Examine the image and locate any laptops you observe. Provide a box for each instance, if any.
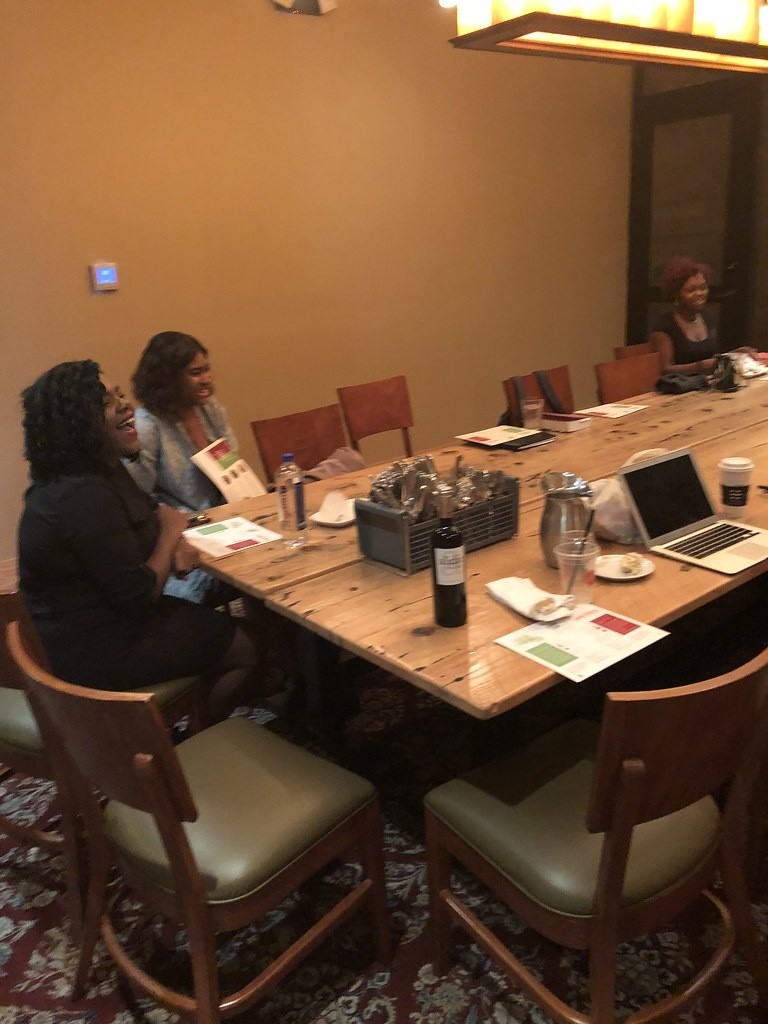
[618,447,768,575]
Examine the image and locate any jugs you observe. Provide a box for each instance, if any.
[539,472,593,569]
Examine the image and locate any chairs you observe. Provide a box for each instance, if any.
[593,352,665,404]
[420,654,768,1023]
[613,342,665,393]
[337,375,418,469]
[248,404,346,494]
[8,606,393,1024]
[501,365,576,430]
[0,592,203,948]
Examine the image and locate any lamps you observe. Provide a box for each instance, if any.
[437,1,767,76]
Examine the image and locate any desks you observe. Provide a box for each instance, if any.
[176,360,767,972]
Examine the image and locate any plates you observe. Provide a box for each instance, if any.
[596,555,655,580]
[309,498,370,528]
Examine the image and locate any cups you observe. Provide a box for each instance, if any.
[717,457,754,517]
[553,542,600,603]
[560,530,593,544]
[521,399,544,430]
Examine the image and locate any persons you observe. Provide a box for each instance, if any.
[650,257,757,376]
[119,329,245,610]
[16,359,254,742]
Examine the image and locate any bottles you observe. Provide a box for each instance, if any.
[276,454,307,547]
[432,492,467,628]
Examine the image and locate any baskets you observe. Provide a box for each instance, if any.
[355,471,520,572]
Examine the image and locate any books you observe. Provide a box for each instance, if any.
[470,424,557,450]
